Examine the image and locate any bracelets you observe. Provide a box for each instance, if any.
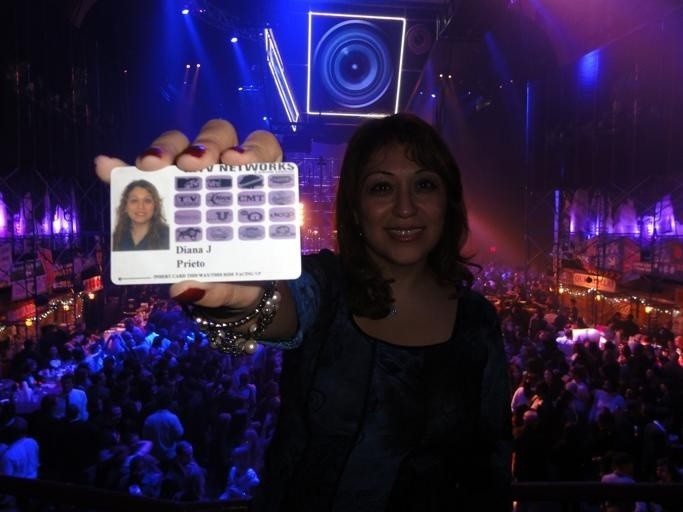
[176,277,282,361]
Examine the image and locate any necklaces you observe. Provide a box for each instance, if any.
[383,278,418,317]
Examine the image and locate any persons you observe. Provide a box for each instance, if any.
[111,169,171,253]
[90,103,518,511]
